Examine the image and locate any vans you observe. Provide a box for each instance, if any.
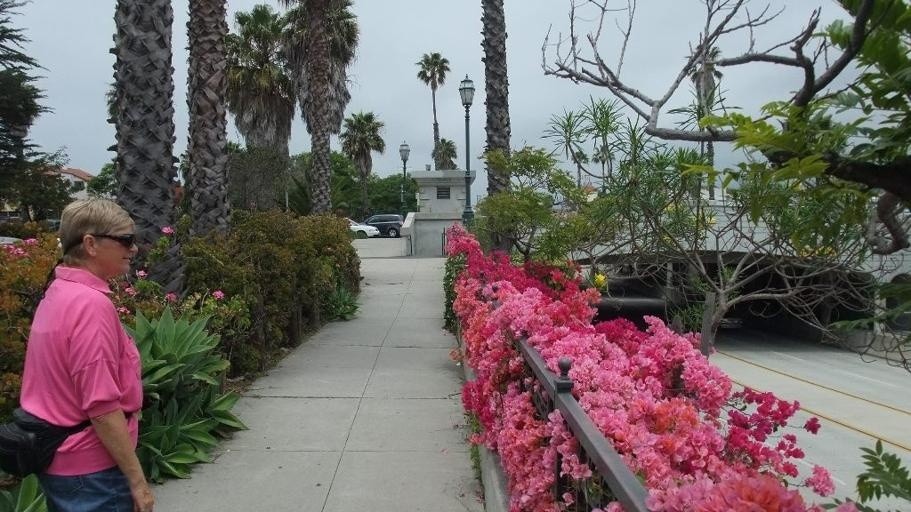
[363,214,405,238]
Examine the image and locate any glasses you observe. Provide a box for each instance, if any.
[80,233,136,248]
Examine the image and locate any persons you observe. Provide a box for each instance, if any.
[20,201,155,512]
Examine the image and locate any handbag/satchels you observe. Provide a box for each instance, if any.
[0,405,70,479]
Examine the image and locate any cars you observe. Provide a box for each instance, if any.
[343,214,380,239]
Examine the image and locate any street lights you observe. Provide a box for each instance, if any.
[460,73,478,235]
[399,139,411,217]
